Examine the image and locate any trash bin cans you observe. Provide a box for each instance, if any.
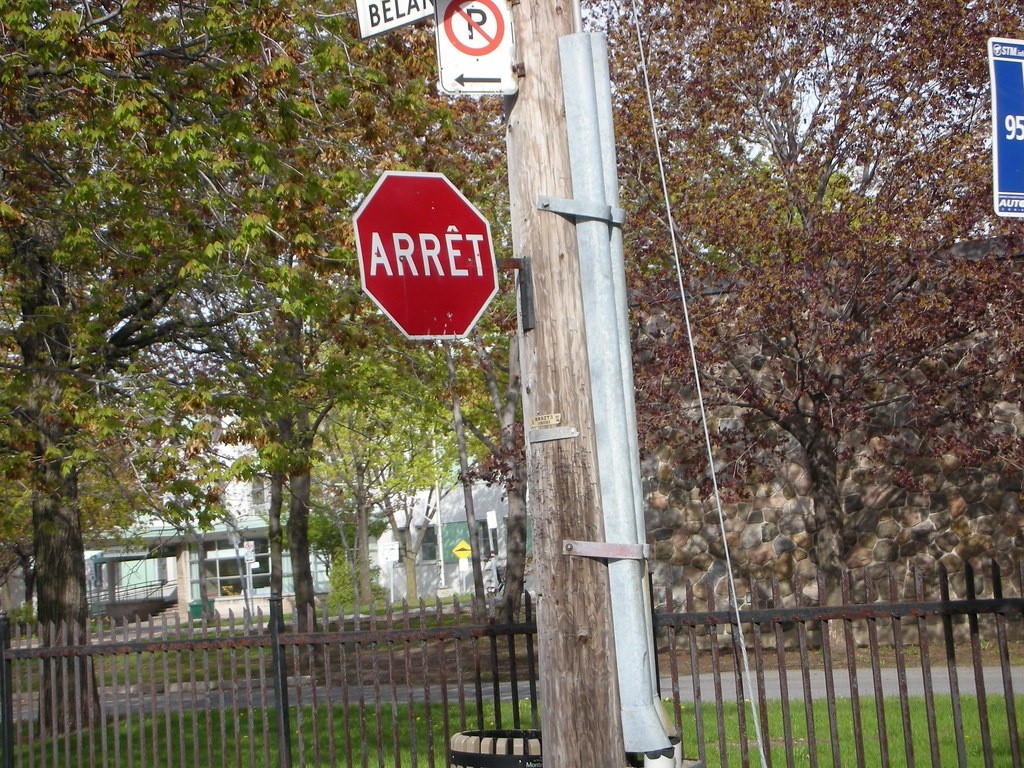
[191,599,215,617]
[449,729,542,768]
